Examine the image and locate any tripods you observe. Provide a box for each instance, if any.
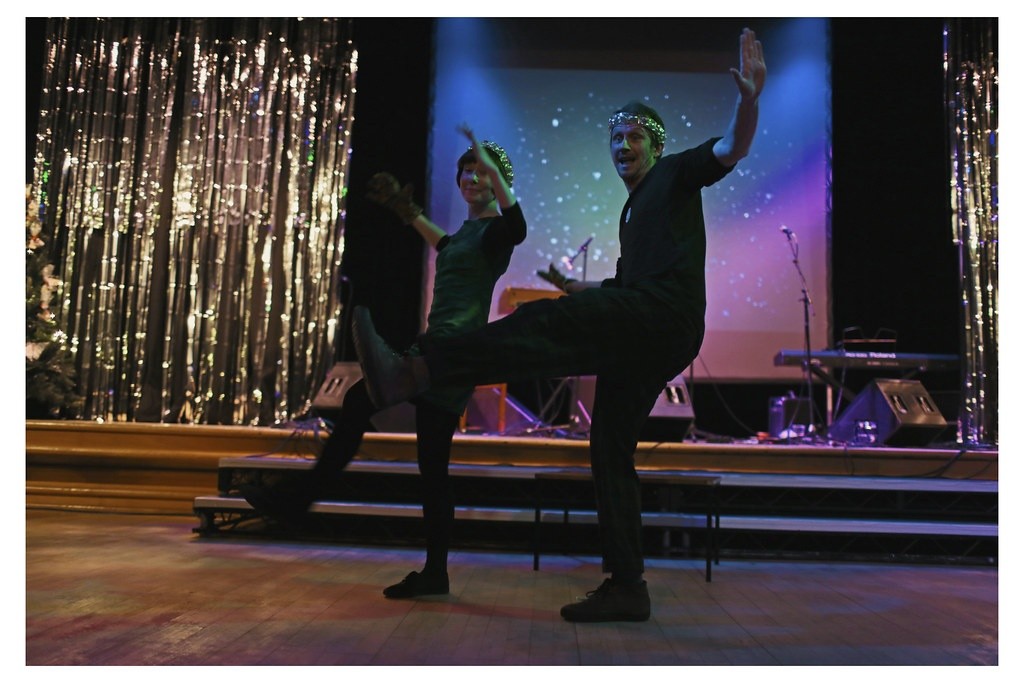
[773,234,854,446]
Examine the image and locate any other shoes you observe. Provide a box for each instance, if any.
[245,474,314,533]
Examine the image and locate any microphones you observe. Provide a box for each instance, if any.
[561,256,573,270]
[779,226,795,237]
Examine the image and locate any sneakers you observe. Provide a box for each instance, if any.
[559,569,652,624]
[351,303,434,411]
[383,567,449,600]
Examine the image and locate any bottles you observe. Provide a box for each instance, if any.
[770,398,784,439]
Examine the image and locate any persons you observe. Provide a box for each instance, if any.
[351,26,768,621]
[247,121,528,599]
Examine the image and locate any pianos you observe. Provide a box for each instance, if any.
[774,350,966,370]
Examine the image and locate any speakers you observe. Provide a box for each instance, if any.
[831,379,948,446]
[574,374,695,443]
[313,361,416,435]
[766,397,808,437]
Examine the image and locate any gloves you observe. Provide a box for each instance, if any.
[537,262,576,295]
[366,174,423,226]
